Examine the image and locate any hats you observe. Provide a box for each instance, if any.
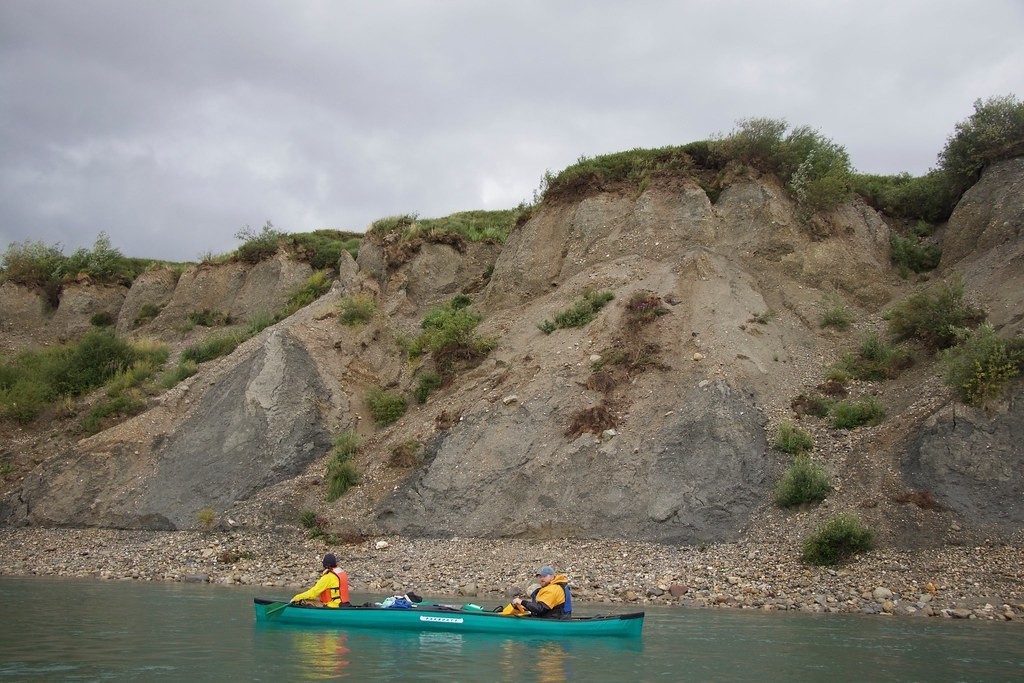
[534,566,556,578]
[322,554,336,567]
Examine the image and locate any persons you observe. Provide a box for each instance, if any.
[512,567,570,617]
[290,553,349,607]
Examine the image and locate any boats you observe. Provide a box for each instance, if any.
[255,597,646,637]
